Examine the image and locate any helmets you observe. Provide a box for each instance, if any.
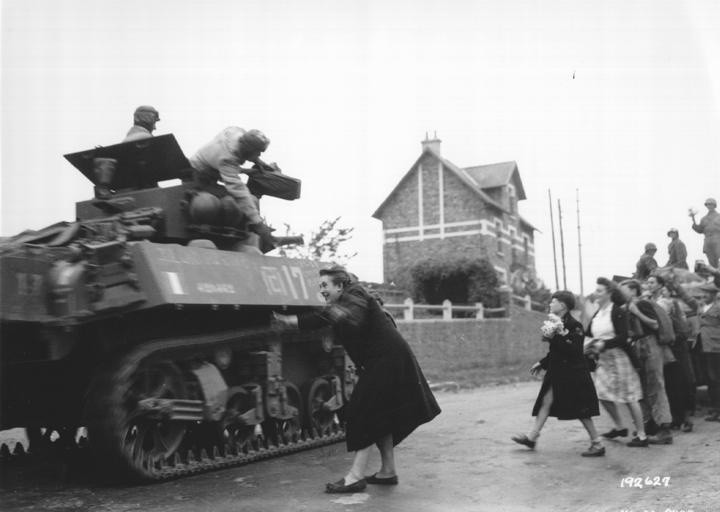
[134,106,160,130]
[705,199,716,206]
[668,229,678,237]
[240,130,268,152]
[646,243,656,252]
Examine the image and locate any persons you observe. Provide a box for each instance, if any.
[666,229,688,270]
[634,243,658,282]
[689,199,720,269]
[182,125,276,242]
[270,265,442,494]
[122,104,161,189]
[580,258,720,448]
[511,290,608,457]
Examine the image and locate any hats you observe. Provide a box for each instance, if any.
[699,282,720,291]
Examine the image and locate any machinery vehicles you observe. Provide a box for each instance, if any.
[1,133,399,484]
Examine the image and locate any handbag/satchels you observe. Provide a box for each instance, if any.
[647,300,676,346]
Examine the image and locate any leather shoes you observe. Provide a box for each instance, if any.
[326,479,366,491]
[366,473,397,484]
[511,407,719,457]
